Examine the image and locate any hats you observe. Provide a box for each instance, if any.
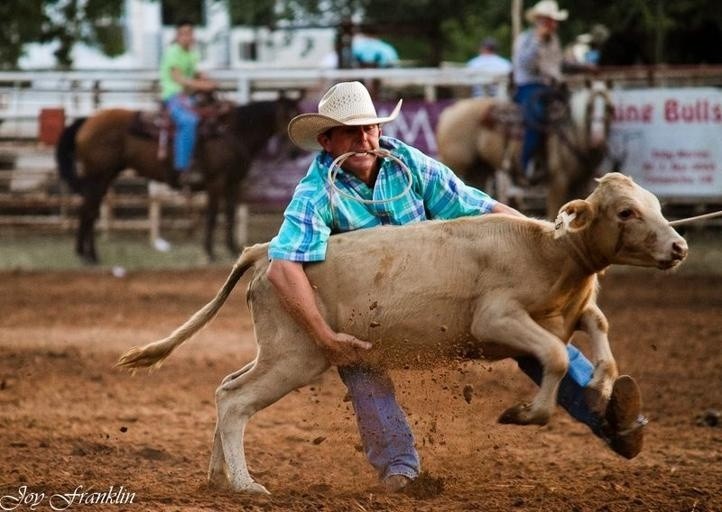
[524,1,569,23]
[287,81,403,152]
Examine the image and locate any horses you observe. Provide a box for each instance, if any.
[56,85,307,265]
[436,75,614,222]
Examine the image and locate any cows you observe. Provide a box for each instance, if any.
[114,171,688,495]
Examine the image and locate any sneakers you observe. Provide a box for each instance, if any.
[602,375,645,459]
[385,474,410,491]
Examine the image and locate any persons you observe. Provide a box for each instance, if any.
[263,80,646,494]
[561,20,611,66]
[158,18,218,179]
[511,0,602,178]
[464,38,509,99]
